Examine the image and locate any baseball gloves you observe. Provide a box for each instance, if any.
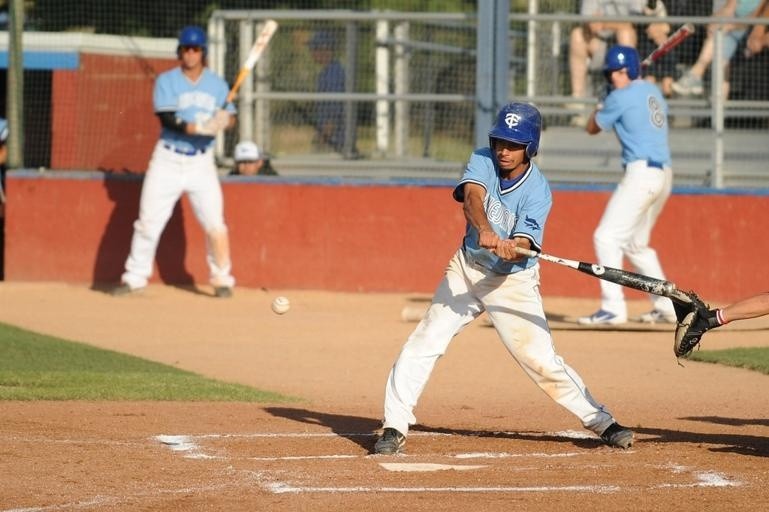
[671,289,711,358]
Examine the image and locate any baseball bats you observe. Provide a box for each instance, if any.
[220,20,277,110]
[640,23,697,70]
[516,248,675,298]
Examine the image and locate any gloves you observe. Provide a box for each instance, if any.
[196,109,229,137]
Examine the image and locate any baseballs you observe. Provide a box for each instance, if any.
[272,297,289,312]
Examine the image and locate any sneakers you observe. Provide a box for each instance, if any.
[111,284,129,296]
[603,422,635,449]
[641,309,672,324]
[216,286,230,298]
[671,73,704,95]
[577,311,628,326]
[374,430,403,455]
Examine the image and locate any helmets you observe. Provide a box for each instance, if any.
[602,45,640,83]
[176,25,207,59]
[489,102,541,159]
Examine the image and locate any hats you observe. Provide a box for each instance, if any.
[234,142,261,163]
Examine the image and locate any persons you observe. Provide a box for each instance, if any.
[300,33,364,162]
[575,44,679,325]
[109,26,236,300]
[372,102,636,453]
[568,0,674,128]
[671,0,769,122]
[674,291,769,358]
